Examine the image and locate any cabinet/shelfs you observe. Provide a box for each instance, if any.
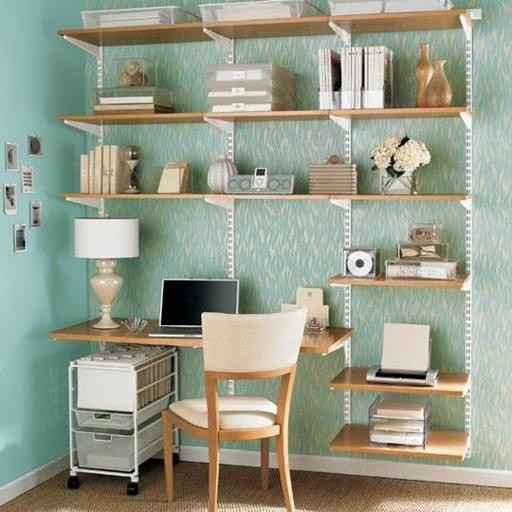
[329,7,484,462]
[56,14,353,210]
[68,350,181,495]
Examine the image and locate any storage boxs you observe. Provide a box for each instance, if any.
[74,394,171,473]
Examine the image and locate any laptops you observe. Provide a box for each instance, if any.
[149,279,240,338]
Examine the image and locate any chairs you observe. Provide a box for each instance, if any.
[162,306,308,512]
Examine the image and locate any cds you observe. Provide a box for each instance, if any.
[347,251,372,277]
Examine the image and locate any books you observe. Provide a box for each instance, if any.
[317,44,396,111]
[133,352,174,411]
[199,2,309,22]
[96,5,186,31]
[157,162,191,194]
[307,162,359,196]
[78,143,133,196]
[368,399,431,449]
[89,85,174,115]
[330,0,456,18]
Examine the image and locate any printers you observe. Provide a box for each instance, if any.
[366,322,438,386]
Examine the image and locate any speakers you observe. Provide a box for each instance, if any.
[225,168,294,195]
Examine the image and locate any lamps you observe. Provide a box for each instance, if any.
[73,216,140,330]
